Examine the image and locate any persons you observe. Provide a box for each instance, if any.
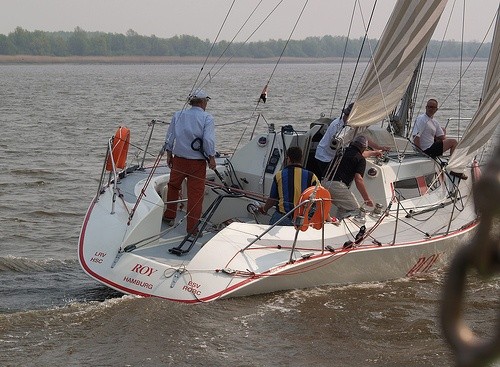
[412,98,469,183]
[323,135,374,219]
[159,92,217,238]
[312,102,392,189]
[259,146,325,228]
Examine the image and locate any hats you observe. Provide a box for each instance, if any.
[193,90,212,99]
[342,103,354,115]
[353,136,368,149]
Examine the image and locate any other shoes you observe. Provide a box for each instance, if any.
[162,217,175,227]
[191,231,203,238]
[449,170,468,180]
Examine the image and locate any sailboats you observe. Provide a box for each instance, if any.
[76,0,500,305]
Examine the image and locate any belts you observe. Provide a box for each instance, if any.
[328,178,350,189]
[277,210,294,219]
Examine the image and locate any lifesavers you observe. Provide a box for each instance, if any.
[106,127,131,170]
[294,185,331,232]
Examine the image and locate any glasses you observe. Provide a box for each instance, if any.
[427,105,437,109]
